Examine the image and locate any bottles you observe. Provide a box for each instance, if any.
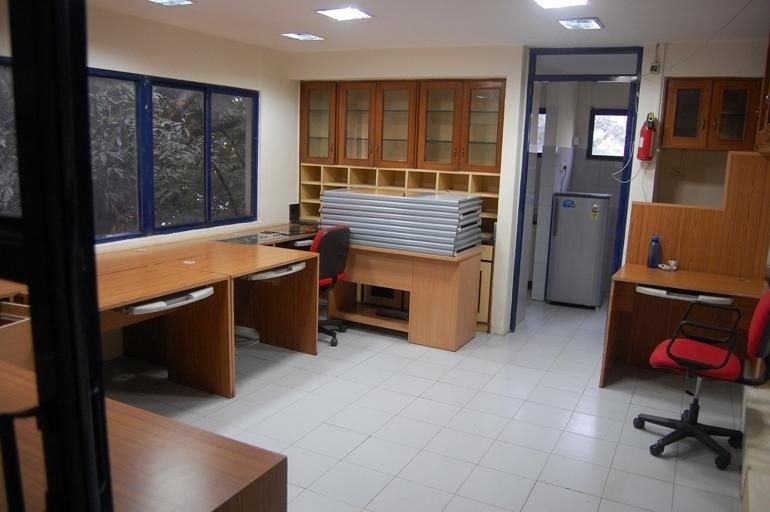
[647,236,660,267]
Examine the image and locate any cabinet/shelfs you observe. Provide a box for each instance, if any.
[300,81,338,167]
[328,244,484,352]
[337,79,506,174]
[298,164,500,332]
[657,76,764,153]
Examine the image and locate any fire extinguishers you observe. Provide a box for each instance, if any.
[637,113,657,160]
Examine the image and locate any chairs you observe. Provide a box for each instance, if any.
[633,290,770,470]
[309,224,350,346]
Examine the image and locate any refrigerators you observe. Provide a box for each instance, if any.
[544,191,616,309]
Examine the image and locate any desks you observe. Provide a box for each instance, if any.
[599,262,769,391]
[95,224,322,400]
